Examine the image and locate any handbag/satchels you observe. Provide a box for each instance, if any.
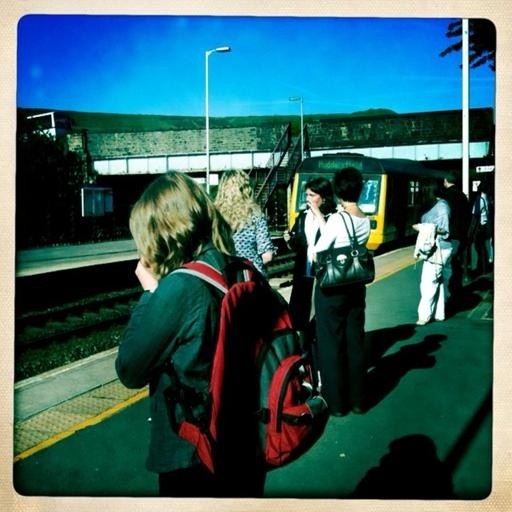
[309,210,377,292]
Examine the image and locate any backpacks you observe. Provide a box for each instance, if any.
[166,258,330,480]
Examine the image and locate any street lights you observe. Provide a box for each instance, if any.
[289,96,304,163]
[205,46,231,195]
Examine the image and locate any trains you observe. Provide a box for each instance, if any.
[288,153,456,257]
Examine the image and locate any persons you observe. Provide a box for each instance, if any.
[284,176,338,392]
[213,169,279,282]
[415,186,457,326]
[116,171,327,497]
[470,179,493,277]
[307,166,374,415]
[443,174,471,291]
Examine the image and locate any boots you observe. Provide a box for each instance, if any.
[347,367,371,417]
[324,374,348,420]
